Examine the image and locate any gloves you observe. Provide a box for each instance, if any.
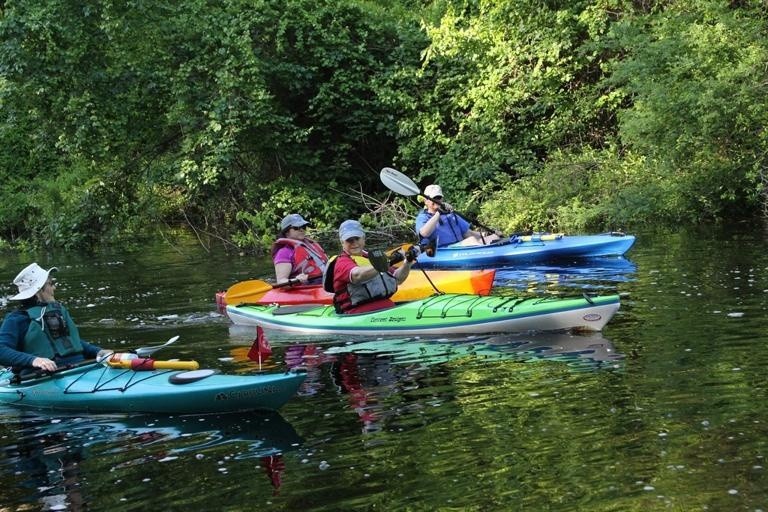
[405,249,416,263]
[390,253,403,267]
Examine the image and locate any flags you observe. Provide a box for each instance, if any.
[245,325,272,365]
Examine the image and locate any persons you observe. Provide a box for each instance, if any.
[0,262,113,381]
[7,406,83,512]
[282,340,337,400]
[415,183,487,248]
[333,349,407,442]
[320,219,420,315]
[271,212,330,291]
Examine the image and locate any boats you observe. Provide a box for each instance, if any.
[393,231,639,269]
[0,355,310,418]
[229,291,623,344]
[216,256,497,312]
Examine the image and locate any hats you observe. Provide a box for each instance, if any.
[10,263,58,300]
[423,185,444,199]
[338,220,364,241]
[280,214,310,229]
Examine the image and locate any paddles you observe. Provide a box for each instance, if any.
[0,334,179,386]
[369,247,416,272]
[379,167,505,237]
[224,273,324,307]
